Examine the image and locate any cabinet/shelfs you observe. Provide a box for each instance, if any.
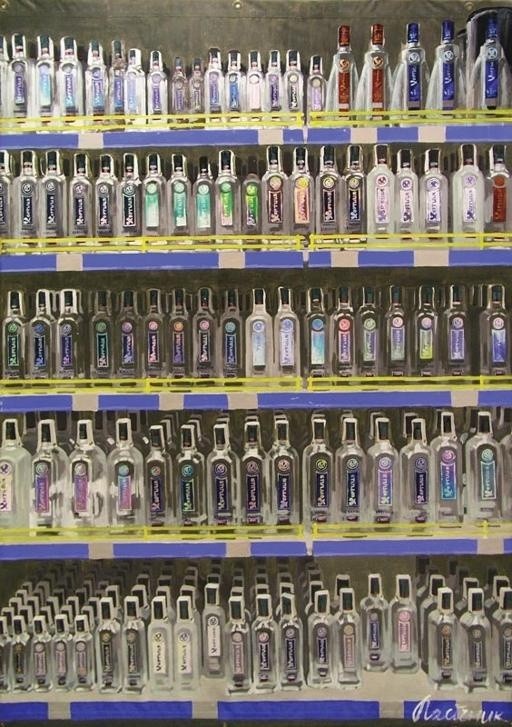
[0,110,512,726]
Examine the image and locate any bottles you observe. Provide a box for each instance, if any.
[1,143,512,255]
[1,405,511,541]
[0,281,511,393]
[1,17,511,123]
[0,555,512,695]
